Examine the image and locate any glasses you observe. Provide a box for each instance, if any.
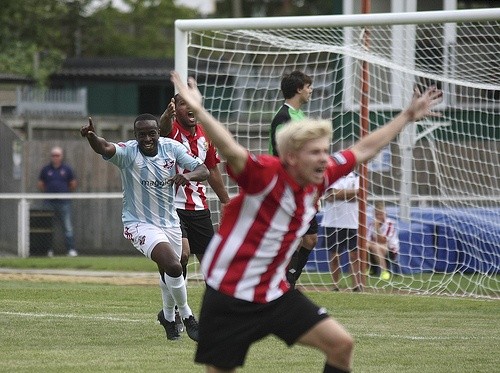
[51,154,60,157]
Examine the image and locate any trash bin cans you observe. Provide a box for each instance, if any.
[30,208,56,256]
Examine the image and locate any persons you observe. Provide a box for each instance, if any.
[269,72,317,291]
[80,114,210,342]
[319,172,363,291]
[158,93,230,279]
[38,147,78,257]
[170,72,442,373]
[364,201,398,281]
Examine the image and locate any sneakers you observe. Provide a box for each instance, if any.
[48,251,53,257]
[174,306,183,331]
[68,250,78,257]
[182,313,199,341]
[379,270,390,280]
[157,307,180,341]
[333,283,364,292]
